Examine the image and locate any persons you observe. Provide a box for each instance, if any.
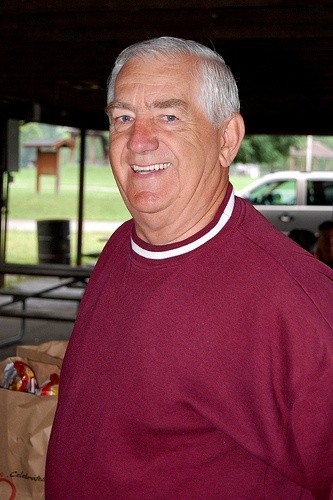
[44,36,333,500]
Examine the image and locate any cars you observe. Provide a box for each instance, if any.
[234,169,333,235]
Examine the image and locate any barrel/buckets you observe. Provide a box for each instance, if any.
[37,219,71,264]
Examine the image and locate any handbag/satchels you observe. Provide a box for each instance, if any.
[0,340,67,500]
